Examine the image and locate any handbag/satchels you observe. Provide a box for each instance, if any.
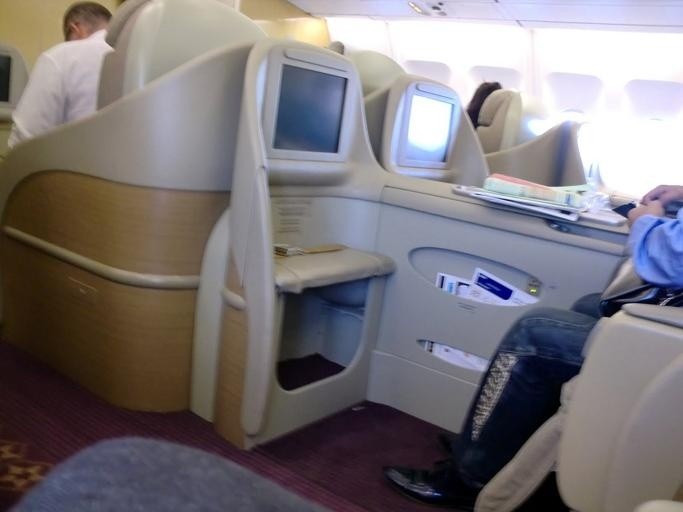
[599,258,683,316]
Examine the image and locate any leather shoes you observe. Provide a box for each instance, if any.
[388,466,477,510]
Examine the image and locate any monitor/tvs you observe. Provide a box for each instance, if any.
[401,93,452,165]
[271,63,349,154]
[0,54,12,105]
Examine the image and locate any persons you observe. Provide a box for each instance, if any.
[380,185,683,512]
[0,0,116,168]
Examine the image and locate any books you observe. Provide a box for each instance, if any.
[451,171,629,227]
